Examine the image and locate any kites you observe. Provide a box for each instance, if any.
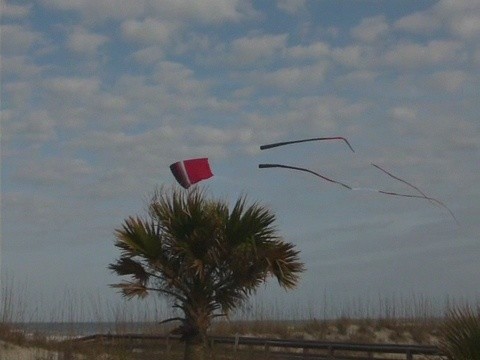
[168,136,459,224]
